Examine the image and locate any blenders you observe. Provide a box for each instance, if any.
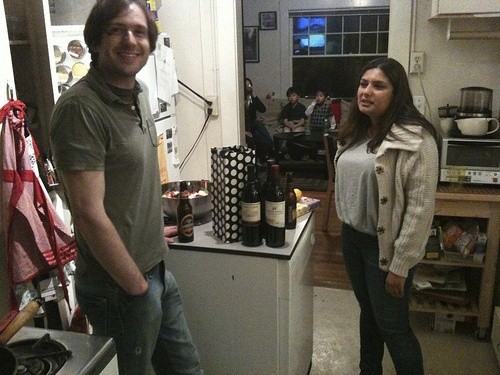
[439,103,458,134]
[457,86,493,132]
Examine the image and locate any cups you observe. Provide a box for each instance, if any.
[456,117,499,136]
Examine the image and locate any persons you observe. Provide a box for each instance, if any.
[244,78,275,152]
[49,0,204,375]
[334,57,438,375]
[305,90,336,133]
[282,86,307,160]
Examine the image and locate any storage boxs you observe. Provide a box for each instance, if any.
[210,145,255,244]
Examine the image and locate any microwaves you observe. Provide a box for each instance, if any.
[436,133,500,185]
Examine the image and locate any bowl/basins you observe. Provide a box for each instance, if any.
[161,180,212,219]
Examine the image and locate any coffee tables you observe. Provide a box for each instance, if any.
[273,132,327,169]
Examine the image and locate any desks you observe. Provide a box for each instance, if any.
[165,210,316,375]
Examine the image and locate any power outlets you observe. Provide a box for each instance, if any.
[412,96,425,115]
[410,52,424,73]
[204,96,218,116]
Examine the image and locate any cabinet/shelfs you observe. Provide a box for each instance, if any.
[413,183,500,340]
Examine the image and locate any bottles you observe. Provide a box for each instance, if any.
[176,181,194,243]
[241,159,296,247]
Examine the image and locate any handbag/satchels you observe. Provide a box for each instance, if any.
[210,144,258,245]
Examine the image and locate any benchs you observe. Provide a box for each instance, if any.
[259,98,352,140]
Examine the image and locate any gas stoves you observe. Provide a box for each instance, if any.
[0,325,119,375]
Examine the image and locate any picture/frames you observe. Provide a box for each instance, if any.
[258,11,277,30]
[243,26,260,63]
